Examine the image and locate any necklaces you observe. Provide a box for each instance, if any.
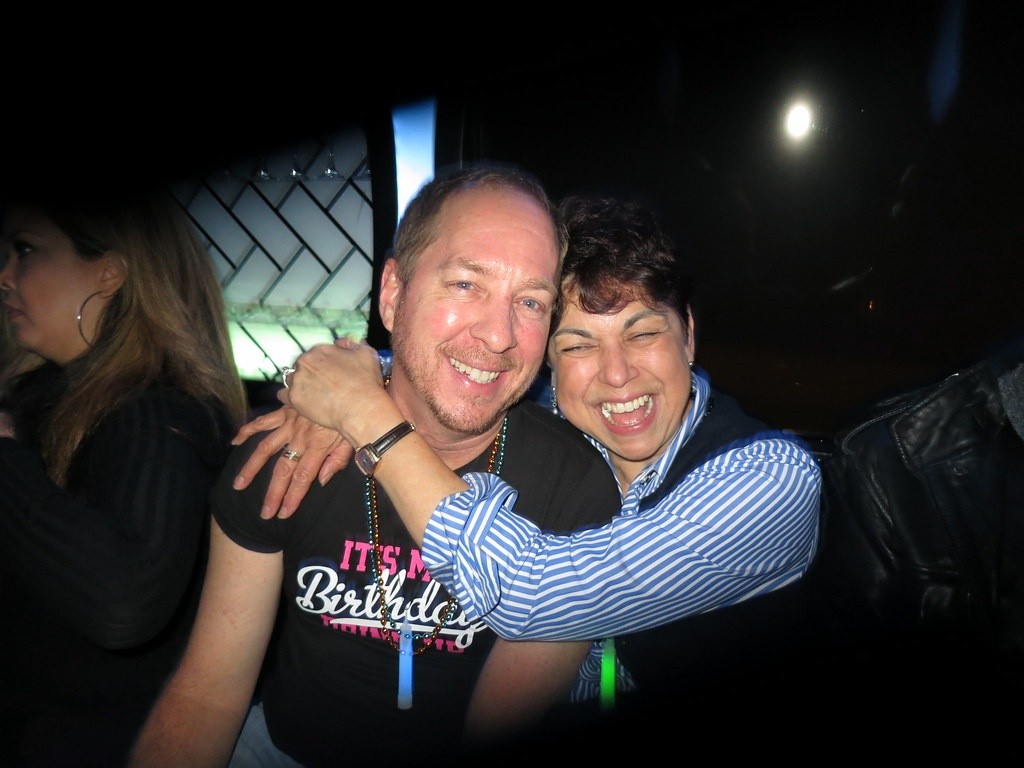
[365,375,507,656]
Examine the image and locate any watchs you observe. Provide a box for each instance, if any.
[354,420,415,476]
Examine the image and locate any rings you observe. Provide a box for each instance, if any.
[281,449,301,463]
[282,367,295,388]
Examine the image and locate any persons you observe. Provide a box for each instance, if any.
[231,192,914,768]
[0,159,251,768]
[130,166,622,768]
